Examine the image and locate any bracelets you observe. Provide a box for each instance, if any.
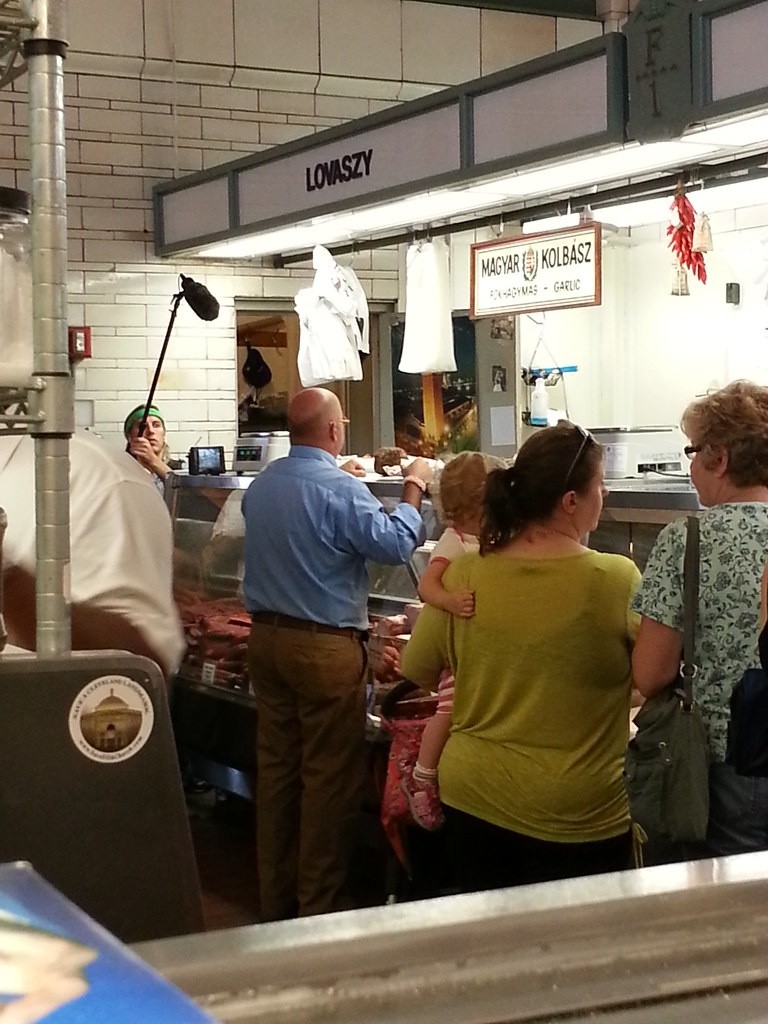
[403,476,426,491]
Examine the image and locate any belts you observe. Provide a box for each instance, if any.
[251,611,369,643]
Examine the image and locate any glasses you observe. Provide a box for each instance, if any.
[684,445,700,459]
[557,418,595,490]
[329,416,350,426]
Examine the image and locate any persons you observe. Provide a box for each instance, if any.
[401,451,509,829]
[400,424,644,901]
[631,379,768,867]
[0,247,185,682]
[241,388,433,924]
[123,405,186,503]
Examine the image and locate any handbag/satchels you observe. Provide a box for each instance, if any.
[623,688,708,845]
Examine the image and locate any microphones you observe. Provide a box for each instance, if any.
[180,274,219,321]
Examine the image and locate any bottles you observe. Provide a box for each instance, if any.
[530,378,549,425]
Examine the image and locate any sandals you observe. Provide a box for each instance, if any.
[401,764,445,831]
